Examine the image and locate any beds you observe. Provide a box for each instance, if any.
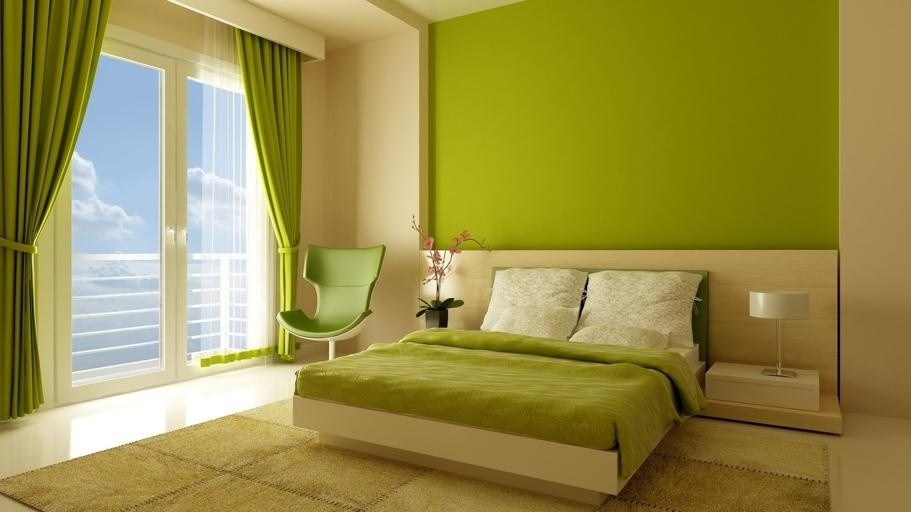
[293,266,709,507]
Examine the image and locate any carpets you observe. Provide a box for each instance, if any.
[0,397,831,512]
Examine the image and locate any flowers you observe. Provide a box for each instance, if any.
[412,215,493,317]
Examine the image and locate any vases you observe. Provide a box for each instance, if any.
[425,310,448,329]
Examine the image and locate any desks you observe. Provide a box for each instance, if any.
[705,361,820,412]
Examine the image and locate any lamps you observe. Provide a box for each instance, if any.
[749,290,809,379]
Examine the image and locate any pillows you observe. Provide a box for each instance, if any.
[480,267,588,331]
[490,300,579,340]
[569,324,673,349]
[573,270,703,348]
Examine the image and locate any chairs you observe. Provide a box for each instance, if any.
[277,245,386,360]
